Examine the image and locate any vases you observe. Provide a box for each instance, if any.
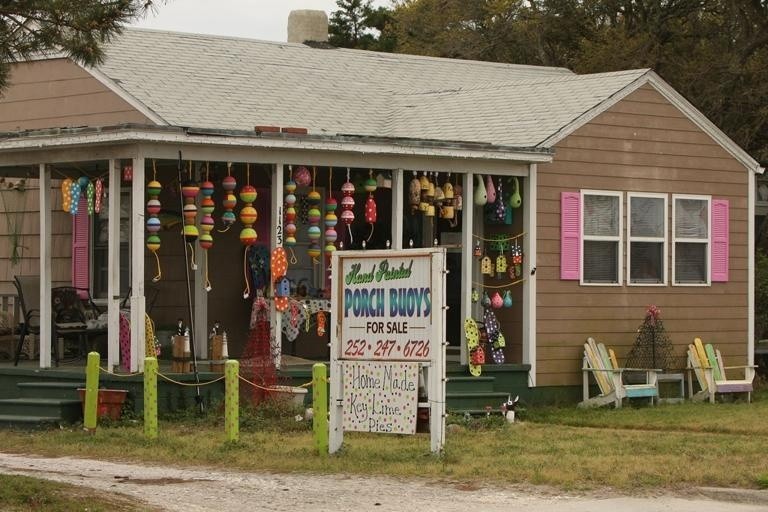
[77,388,129,421]
[265,385,308,406]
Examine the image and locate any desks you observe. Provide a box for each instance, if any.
[249,298,331,361]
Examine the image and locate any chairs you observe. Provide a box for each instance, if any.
[684,337,758,405]
[576,337,663,410]
[13,274,160,368]
